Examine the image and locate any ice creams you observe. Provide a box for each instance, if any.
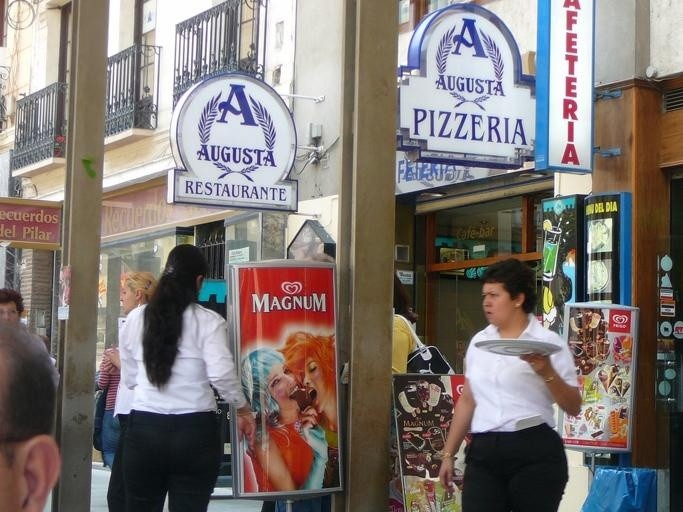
[422,478,437,512]
[598,336,634,401]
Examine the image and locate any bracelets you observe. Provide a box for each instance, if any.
[235,411,251,417]
[438,451,453,460]
[543,373,560,382]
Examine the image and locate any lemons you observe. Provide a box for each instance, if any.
[542,220,553,230]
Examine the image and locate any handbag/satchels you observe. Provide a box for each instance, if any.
[407,346,451,375]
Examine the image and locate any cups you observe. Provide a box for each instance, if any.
[543,226,562,282]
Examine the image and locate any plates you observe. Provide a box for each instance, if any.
[474,339,563,358]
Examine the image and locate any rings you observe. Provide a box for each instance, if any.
[529,361,536,365]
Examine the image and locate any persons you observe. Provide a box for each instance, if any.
[0,286,23,325]
[105,272,160,510]
[278,330,339,489]
[239,349,329,491]
[0,315,61,510]
[438,257,582,512]
[96,345,122,469]
[119,242,257,510]
[391,272,419,377]
